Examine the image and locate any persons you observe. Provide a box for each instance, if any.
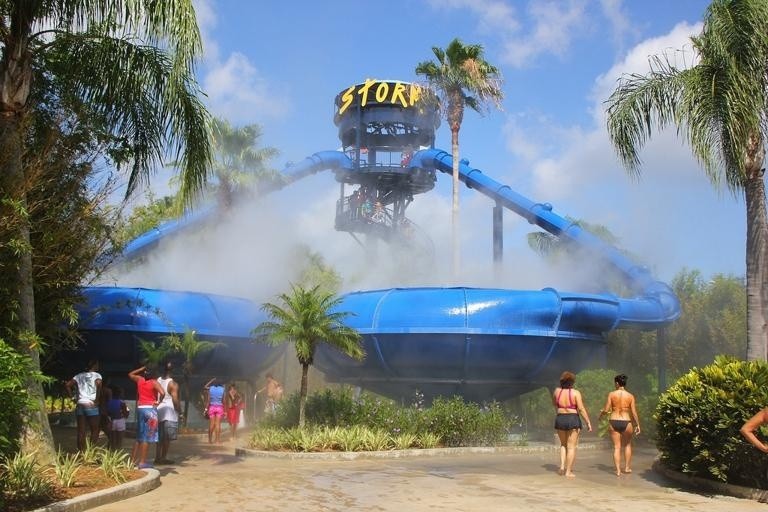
[199,373,286,445]
[598,375,641,477]
[65,355,184,467]
[349,186,372,220]
[552,371,592,477]
[740,406,768,453]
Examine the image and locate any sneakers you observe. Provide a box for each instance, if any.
[154,458,174,464]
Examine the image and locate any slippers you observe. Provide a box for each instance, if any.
[138,464,153,469]
